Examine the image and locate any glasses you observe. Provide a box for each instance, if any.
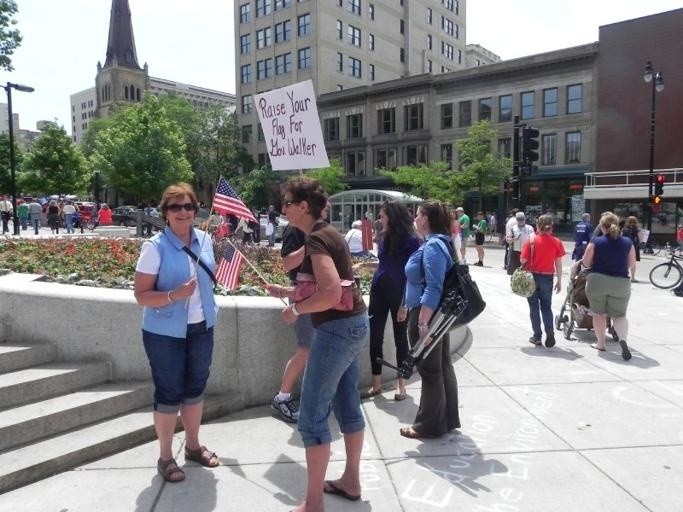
[282,200,299,209]
[164,203,196,212]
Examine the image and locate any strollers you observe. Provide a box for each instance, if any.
[554,257,622,344]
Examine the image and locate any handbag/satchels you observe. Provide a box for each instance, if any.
[509,268,538,298]
[292,272,356,312]
[424,264,486,332]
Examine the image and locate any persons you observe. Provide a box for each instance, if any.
[676,225,683,256]
[1,193,114,233]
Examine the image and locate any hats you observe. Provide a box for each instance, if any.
[515,212,525,219]
[455,206,464,213]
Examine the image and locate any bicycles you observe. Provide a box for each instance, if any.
[647,241,683,290]
[635,234,662,257]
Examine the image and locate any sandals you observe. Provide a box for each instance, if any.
[399,426,442,439]
[185,443,219,468]
[360,384,382,400]
[394,386,407,400]
[155,458,186,483]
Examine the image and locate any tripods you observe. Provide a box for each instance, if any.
[376,290,469,379]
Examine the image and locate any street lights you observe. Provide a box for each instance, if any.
[4,79,39,236]
[641,58,664,257]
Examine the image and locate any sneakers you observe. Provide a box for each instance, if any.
[529,334,631,361]
[629,278,640,284]
[459,258,484,267]
[270,392,301,425]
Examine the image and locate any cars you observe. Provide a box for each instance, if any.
[193,204,290,247]
[0,194,163,229]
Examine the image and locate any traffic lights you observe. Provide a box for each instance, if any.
[654,172,666,197]
[525,124,540,163]
[652,196,662,204]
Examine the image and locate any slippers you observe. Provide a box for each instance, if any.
[319,479,361,501]
